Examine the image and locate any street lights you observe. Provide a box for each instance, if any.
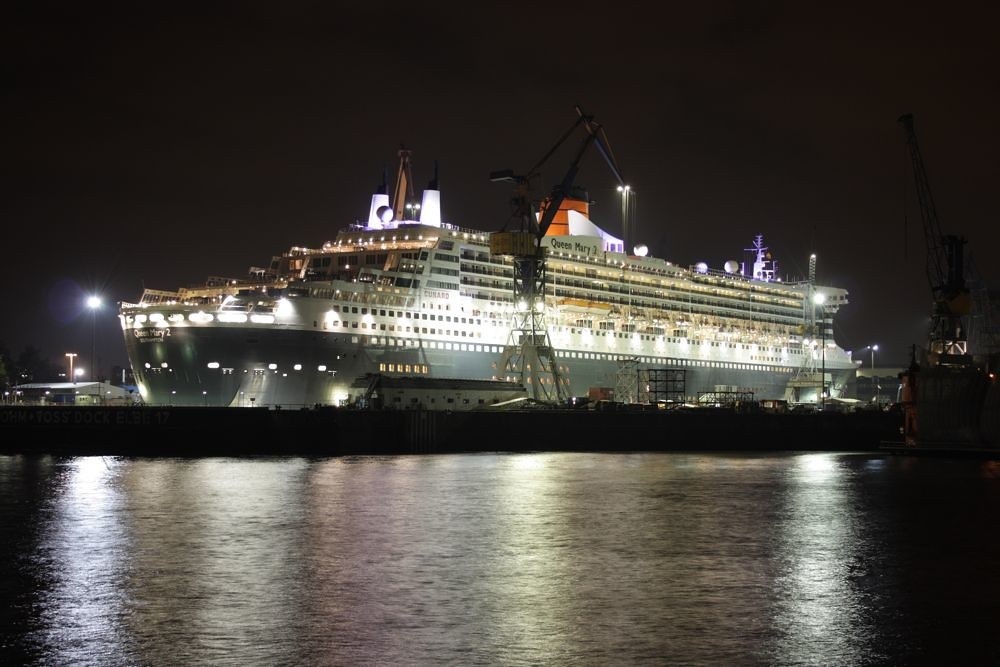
[867,344,879,398]
[65,353,77,382]
[616,185,630,240]
[814,293,825,412]
[87,297,100,381]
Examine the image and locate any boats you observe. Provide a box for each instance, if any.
[365,370,530,413]
[556,298,622,318]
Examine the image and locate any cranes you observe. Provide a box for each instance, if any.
[895,110,991,444]
[489,113,601,409]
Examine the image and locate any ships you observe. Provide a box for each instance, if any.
[116,103,863,412]
